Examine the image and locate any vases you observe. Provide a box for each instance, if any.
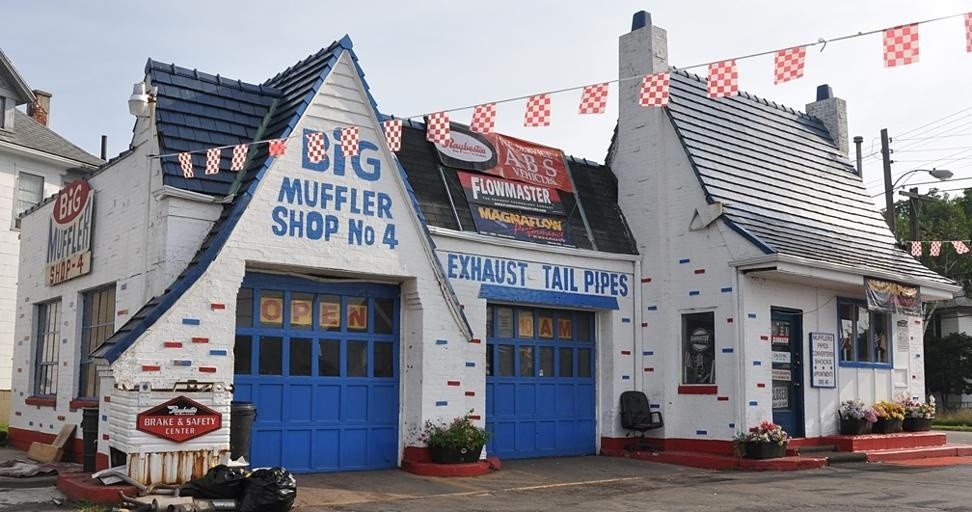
[872,418,901,434]
[740,441,786,459]
[840,419,872,435]
[903,418,932,431]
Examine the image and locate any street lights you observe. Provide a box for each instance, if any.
[882,167,954,249]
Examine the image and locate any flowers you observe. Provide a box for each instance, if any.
[873,400,904,419]
[732,420,791,457]
[898,395,936,418]
[839,399,879,422]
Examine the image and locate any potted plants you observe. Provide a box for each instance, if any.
[405,408,492,464]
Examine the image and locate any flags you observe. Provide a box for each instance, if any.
[707,59,738,98]
[269,126,359,163]
[883,24,919,67]
[774,46,806,84]
[177,143,249,178]
[384,103,495,151]
[525,83,608,126]
[963,13,972,53]
[638,73,670,109]
[911,240,969,256]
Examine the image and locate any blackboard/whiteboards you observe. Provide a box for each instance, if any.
[809,332,836,388]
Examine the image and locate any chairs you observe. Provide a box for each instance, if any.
[619,391,665,452]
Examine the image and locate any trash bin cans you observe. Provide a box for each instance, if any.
[231,401,257,463]
[81,408,99,472]
